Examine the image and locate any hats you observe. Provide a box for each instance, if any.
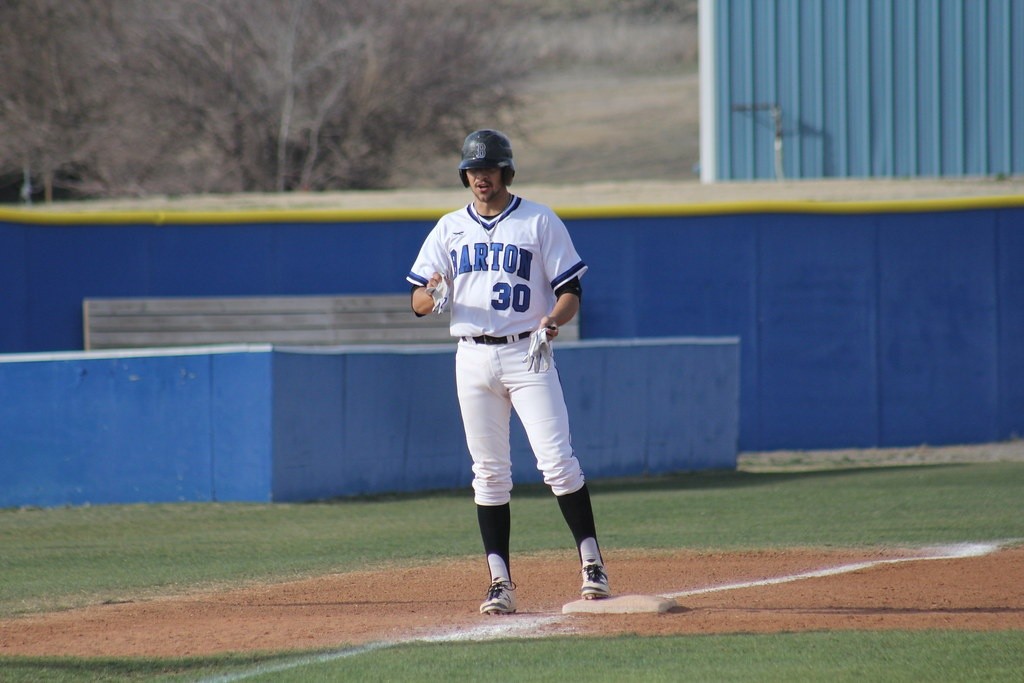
[449,132,527,184]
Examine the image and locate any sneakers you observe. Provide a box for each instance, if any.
[582,554,609,607]
[477,573,535,619]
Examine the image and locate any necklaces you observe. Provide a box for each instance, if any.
[474,193,512,246]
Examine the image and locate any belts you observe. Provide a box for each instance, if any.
[457,337,538,346]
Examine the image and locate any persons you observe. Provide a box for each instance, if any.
[406,131,610,618]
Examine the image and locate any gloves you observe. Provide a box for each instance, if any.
[427,272,451,313]
[525,333,556,375]
